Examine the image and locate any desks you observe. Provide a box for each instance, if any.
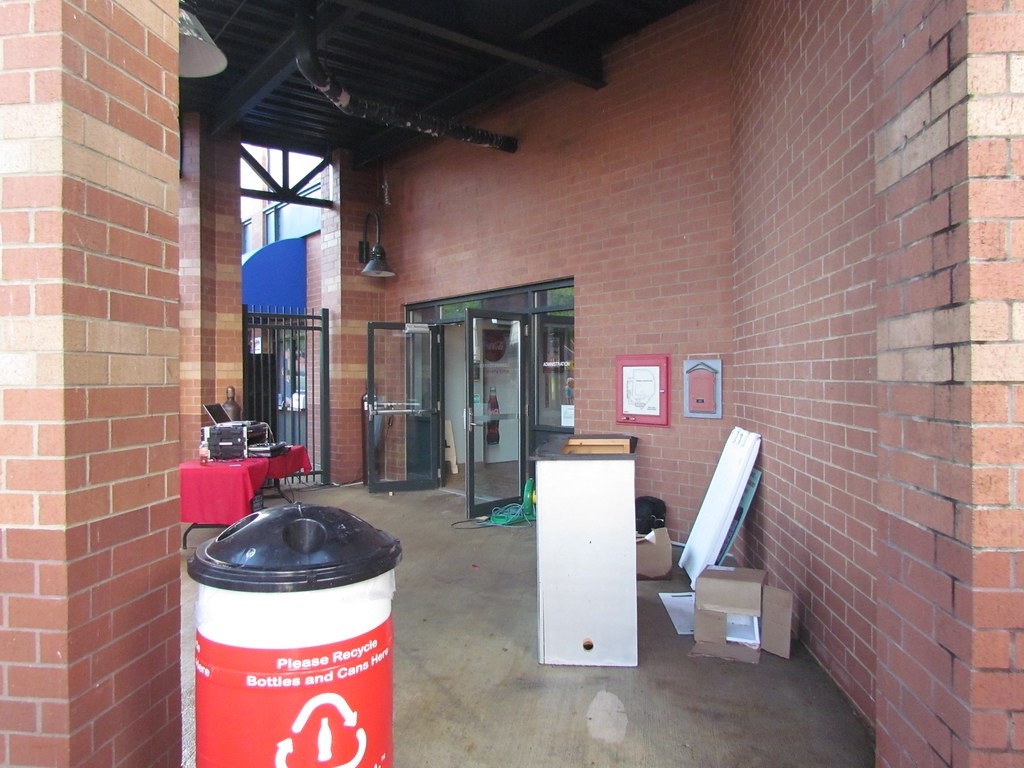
[533,435,640,667]
[180,445,310,550]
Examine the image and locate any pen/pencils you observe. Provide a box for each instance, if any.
[229,465,241,466]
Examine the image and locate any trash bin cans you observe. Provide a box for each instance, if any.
[187,501,406,768]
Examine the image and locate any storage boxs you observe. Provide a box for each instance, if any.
[688,564,794,665]
[637,527,674,581]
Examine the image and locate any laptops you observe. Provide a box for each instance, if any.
[203,402,258,426]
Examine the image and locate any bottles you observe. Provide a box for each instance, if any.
[199,441,208,466]
[487,387,499,445]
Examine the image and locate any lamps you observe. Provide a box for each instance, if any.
[358,208,396,277]
[178,0,229,78]
